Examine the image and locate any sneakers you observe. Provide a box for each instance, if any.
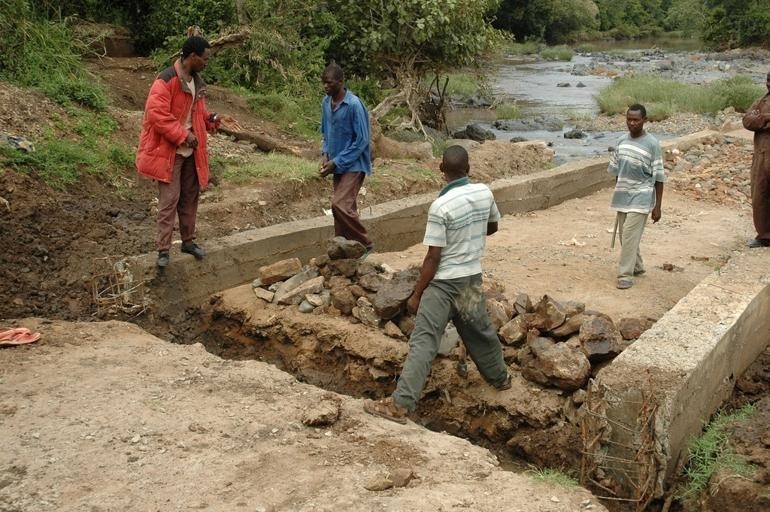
[157,253,170,267]
[616,275,634,289]
[746,238,770,248]
[634,264,646,275]
[181,242,206,257]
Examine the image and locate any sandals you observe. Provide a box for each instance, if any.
[364,397,408,425]
[501,373,512,390]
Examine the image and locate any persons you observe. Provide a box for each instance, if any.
[743,73,769,250]
[363,145,513,426]
[317,62,375,248]
[134,35,241,269]
[608,104,664,290]
